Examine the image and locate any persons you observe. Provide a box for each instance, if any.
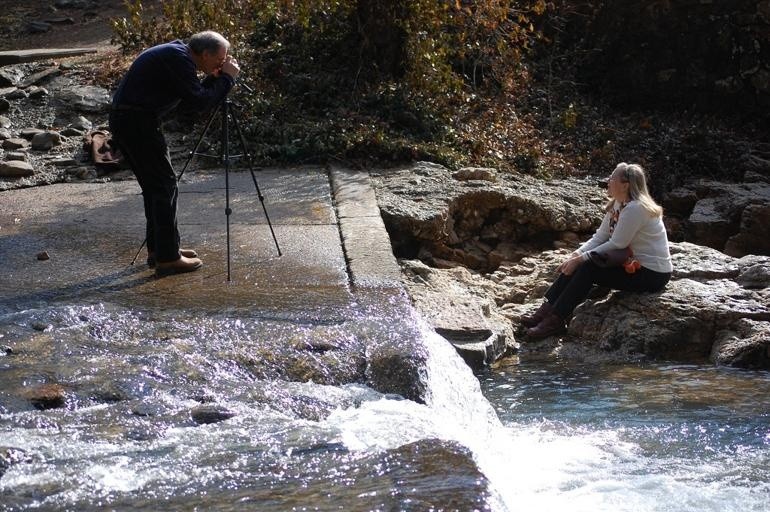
[520,161,674,338]
[107,26,242,277]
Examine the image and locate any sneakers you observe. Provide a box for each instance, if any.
[153,252,203,279]
[521,302,551,328]
[147,247,198,267]
[526,313,567,339]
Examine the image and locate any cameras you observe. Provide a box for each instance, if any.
[219,59,249,83]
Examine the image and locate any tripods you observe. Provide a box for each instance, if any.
[132,97,283,280]
